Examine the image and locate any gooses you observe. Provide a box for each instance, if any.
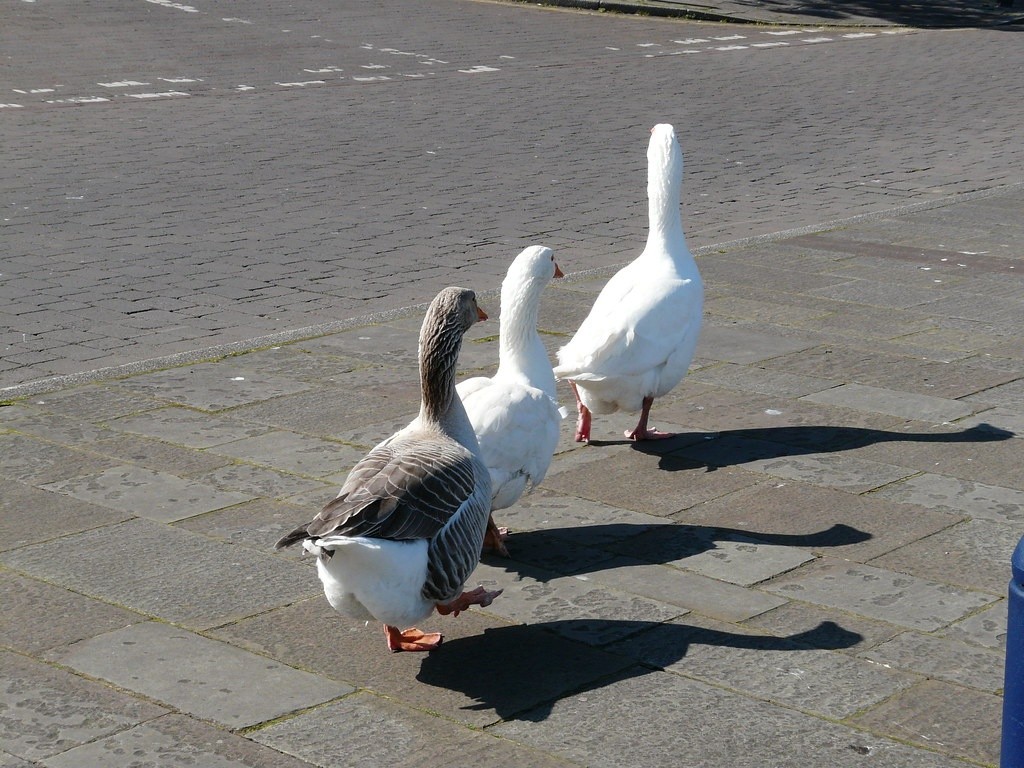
[273,285,504,653]
[553,122,706,444]
[455,244,566,561]
